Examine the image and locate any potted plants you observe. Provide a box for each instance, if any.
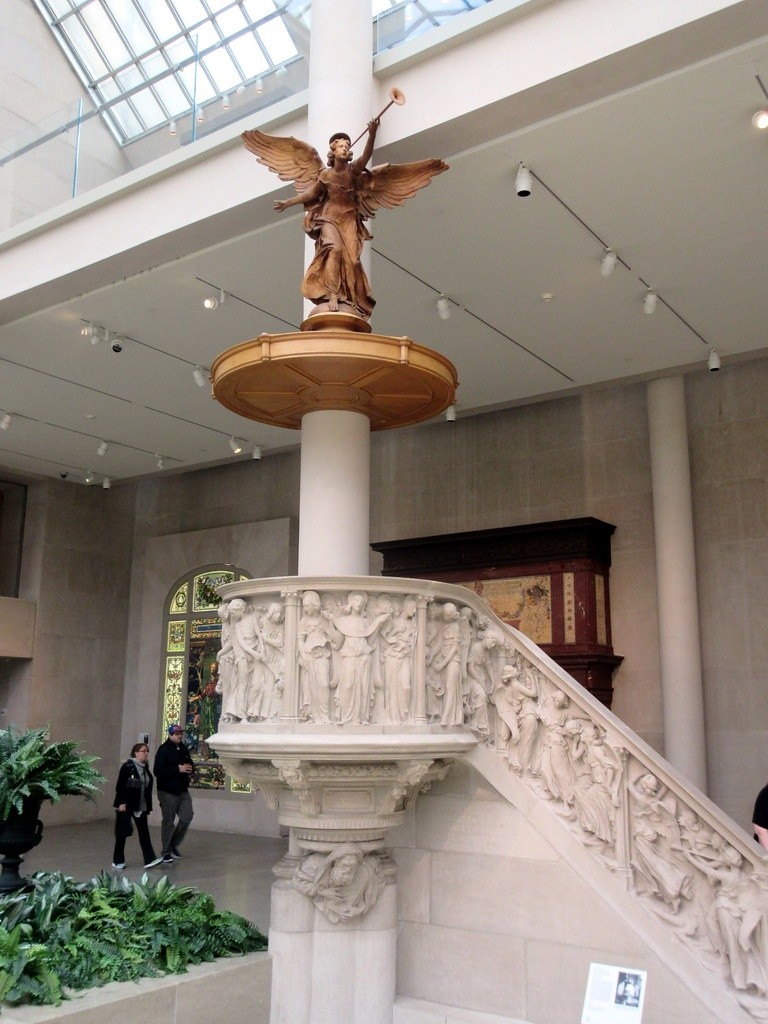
[0,717,109,895]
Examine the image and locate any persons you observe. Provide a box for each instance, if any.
[215,590,768,1018]
[273,116,380,312]
[153,725,194,863]
[188,663,219,758]
[112,743,163,869]
[752,783,768,852]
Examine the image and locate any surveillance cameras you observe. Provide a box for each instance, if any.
[60,472,67,478]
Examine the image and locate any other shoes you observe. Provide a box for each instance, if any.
[144,856,164,869]
[163,854,174,863]
[112,862,125,869]
[168,845,183,859]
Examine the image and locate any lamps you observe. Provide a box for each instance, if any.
[642,287,660,314]
[86,472,96,483]
[201,290,229,313]
[79,321,109,344]
[110,331,126,354]
[96,440,109,457]
[252,444,261,460]
[513,162,533,197]
[192,365,209,388]
[445,406,458,422]
[102,476,111,489]
[0,413,14,431]
[599,248,617,277]
[229,436,243,455]
[435,293,452,319]
[707,349,722,372]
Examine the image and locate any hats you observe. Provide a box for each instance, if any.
[170,725,185,734]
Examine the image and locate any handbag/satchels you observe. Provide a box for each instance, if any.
[115,809,133,838]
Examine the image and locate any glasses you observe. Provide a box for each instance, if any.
[139,750,149,753]
